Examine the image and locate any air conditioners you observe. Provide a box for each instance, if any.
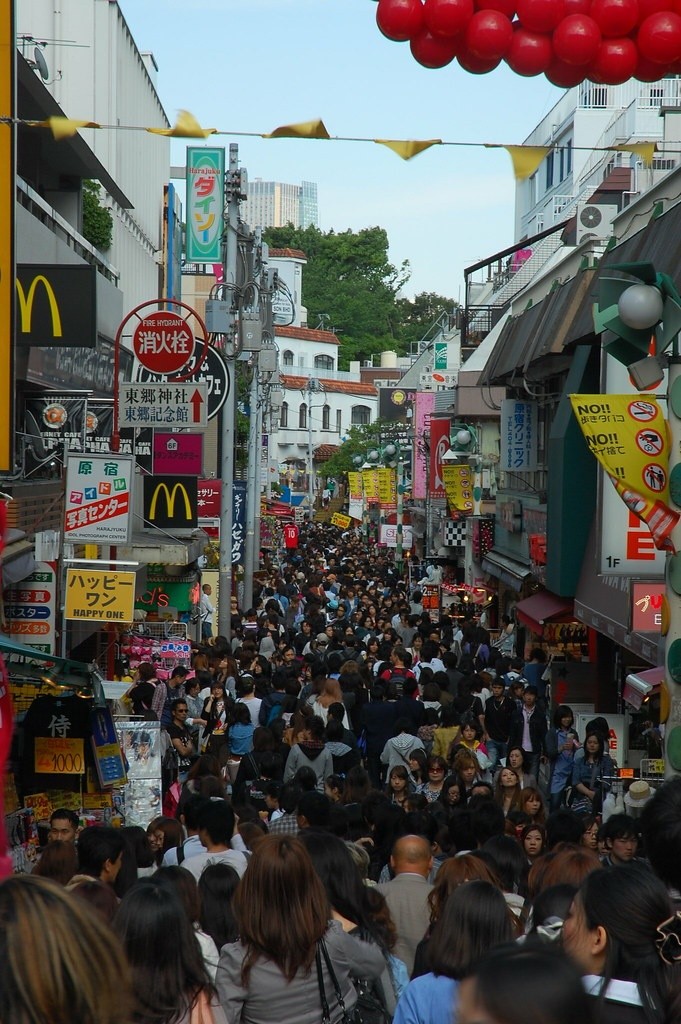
[418,341,430,351]
[389,379,399,387]
[374,379,388,388]
[576,203,619,246]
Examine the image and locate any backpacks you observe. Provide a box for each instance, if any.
[265,693,294,728]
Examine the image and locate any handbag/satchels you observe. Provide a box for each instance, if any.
[315,937,392,1024]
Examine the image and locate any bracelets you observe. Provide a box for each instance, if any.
[562,745,565,749]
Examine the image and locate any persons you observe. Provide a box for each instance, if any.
[0,476,681,1024]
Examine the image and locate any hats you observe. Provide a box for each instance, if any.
[623,780,657,808]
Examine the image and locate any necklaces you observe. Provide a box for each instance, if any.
[431,784,442,789]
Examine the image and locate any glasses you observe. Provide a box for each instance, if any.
[175,709,189,714]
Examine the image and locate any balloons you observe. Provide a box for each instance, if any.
[373,0,681,89]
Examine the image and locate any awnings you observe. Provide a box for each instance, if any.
[623,665,666,711]
[261,497,291,515]
[516,592,574,637]
